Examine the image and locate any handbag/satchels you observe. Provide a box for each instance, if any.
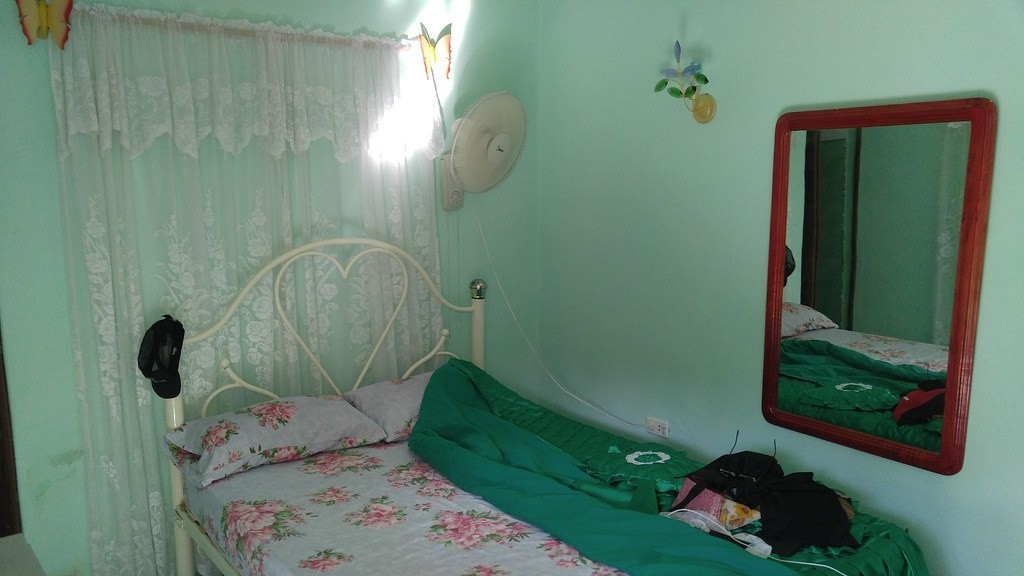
[665,450,788,511]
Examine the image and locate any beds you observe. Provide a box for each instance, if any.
[159,238,910,576]
[776,329,951,456]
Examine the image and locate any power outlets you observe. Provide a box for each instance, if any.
[646,416,669,438]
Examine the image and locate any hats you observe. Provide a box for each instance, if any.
[782,245,796,288]
[138,315,185,399]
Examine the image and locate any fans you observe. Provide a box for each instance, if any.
[440,91,524,211]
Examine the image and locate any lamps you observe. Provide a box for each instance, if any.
[653,41,716,123]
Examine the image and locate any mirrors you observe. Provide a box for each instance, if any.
[761,97,998,477]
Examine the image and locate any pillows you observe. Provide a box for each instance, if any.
[342,371,439,443]
[781,302,839,337]
[163,393,386,488]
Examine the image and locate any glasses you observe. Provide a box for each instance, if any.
[719,430,776,484]
